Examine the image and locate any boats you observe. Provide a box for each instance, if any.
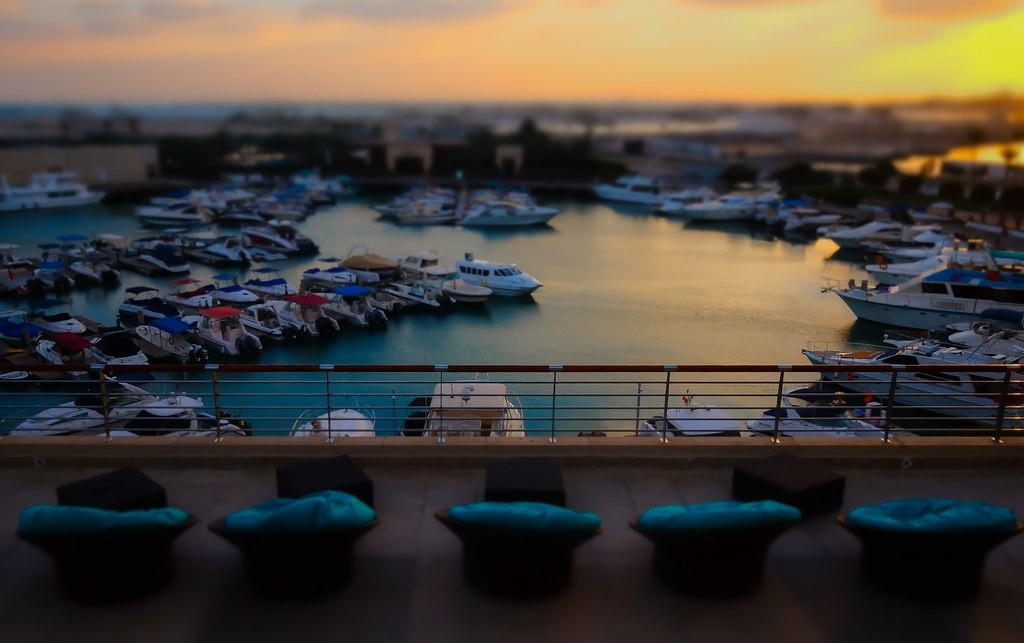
[0,160,563,379]
[591,166,1023,430]
[0,170,110,213]
[289,395,377,437]
[638,389,744,438]
[745,384,892,437]
[455,252,542,298]
[401,371,527,438]
[6,379,160,437]
[97,392,233,427]
